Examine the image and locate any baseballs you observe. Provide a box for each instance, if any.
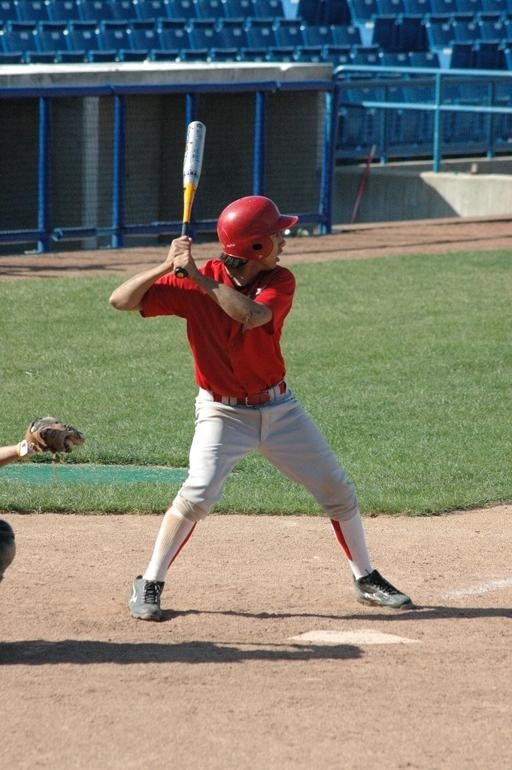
[64,438,74,452]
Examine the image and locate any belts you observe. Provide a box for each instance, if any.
[196,379,286,408]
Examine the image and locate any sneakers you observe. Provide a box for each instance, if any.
[352,566,414,611]
[128,574,164,622]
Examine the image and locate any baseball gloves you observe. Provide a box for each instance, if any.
[24,417,83,454]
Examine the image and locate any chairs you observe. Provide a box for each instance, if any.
[0,0,512,159]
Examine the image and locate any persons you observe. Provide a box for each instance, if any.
[107,195,415,618]
[1,414,90,580]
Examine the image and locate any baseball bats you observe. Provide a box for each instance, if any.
[347,144,378,221]
[174,120,207,277]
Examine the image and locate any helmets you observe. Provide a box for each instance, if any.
[217,195,300,264]
